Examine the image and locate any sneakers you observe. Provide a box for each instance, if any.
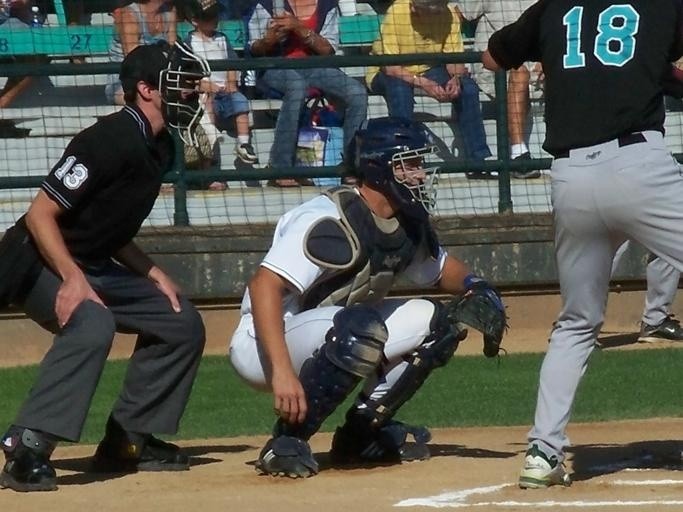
[231,143,258,164]
[637,317,682,344]
[91,435,189,471]
[255,439,319,479]
[518,443,566,489]
[0,453,57,491]
[509,152,541,178]
[329,425,430,469]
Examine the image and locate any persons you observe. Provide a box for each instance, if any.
[0,42,212,492]
[227,114,508,479]
[102,1,543,188]
[549,254,682,349]
[483,1,683,489]
[0,0,53,135]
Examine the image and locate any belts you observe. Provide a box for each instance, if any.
[553,133,646,159]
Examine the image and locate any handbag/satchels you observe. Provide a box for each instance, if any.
[293,94,344,186]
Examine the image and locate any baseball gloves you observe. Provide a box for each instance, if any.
[447,274,508,357]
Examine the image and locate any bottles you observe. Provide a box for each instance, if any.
[29,6,42,31]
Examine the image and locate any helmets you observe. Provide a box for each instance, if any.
[119,39,210,148]
[346,116,443,217]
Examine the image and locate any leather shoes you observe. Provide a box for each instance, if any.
[267,178,298,187]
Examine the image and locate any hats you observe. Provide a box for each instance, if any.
[183,0,226,19]
